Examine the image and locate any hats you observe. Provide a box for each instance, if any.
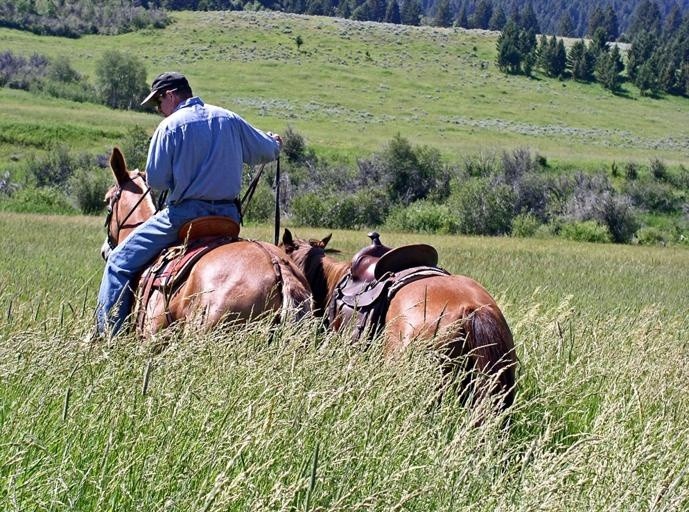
[139,71,188,107]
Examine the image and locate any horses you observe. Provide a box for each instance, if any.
[98,146,314,358]
[278,230,517,427]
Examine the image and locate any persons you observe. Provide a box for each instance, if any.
[85,72,282,340]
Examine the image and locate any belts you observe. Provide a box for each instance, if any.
[206,199,235,205]
